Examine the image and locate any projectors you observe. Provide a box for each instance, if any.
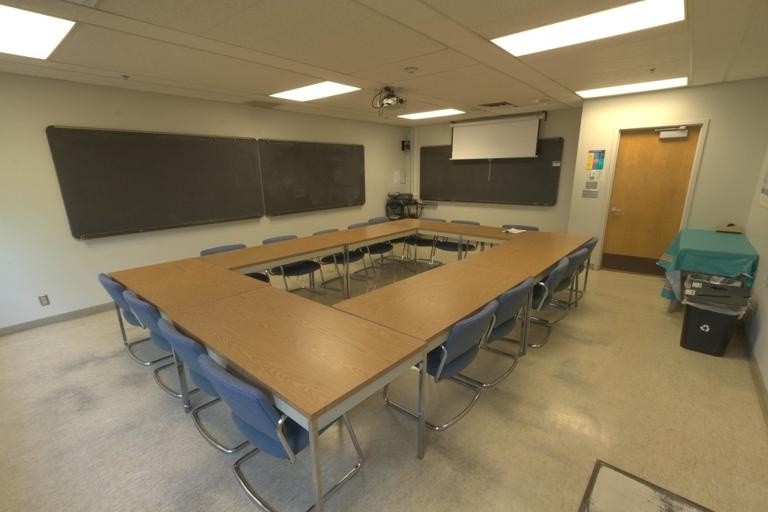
[375,96,408,108]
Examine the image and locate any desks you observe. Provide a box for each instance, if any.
[202,220,417,277]
[330,230,597,453]
[105,254,429,512]
[669,228,759,316]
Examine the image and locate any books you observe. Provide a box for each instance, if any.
[505,228,527,234]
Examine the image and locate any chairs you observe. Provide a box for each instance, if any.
[501,236,597,349]
[201,244,270,287]
[99,273,147,346]
[122,288,173,367]
[156,317,253,456]
[457,276,535,387]
[312,228,369,291]
[349,215,540,269]
[263,234,326,298]
[196,355,366,510]
[382,298,499,431]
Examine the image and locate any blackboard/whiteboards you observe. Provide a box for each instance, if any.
[420,139,561,206]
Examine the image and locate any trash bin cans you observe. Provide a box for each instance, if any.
[680,299,750,356]
